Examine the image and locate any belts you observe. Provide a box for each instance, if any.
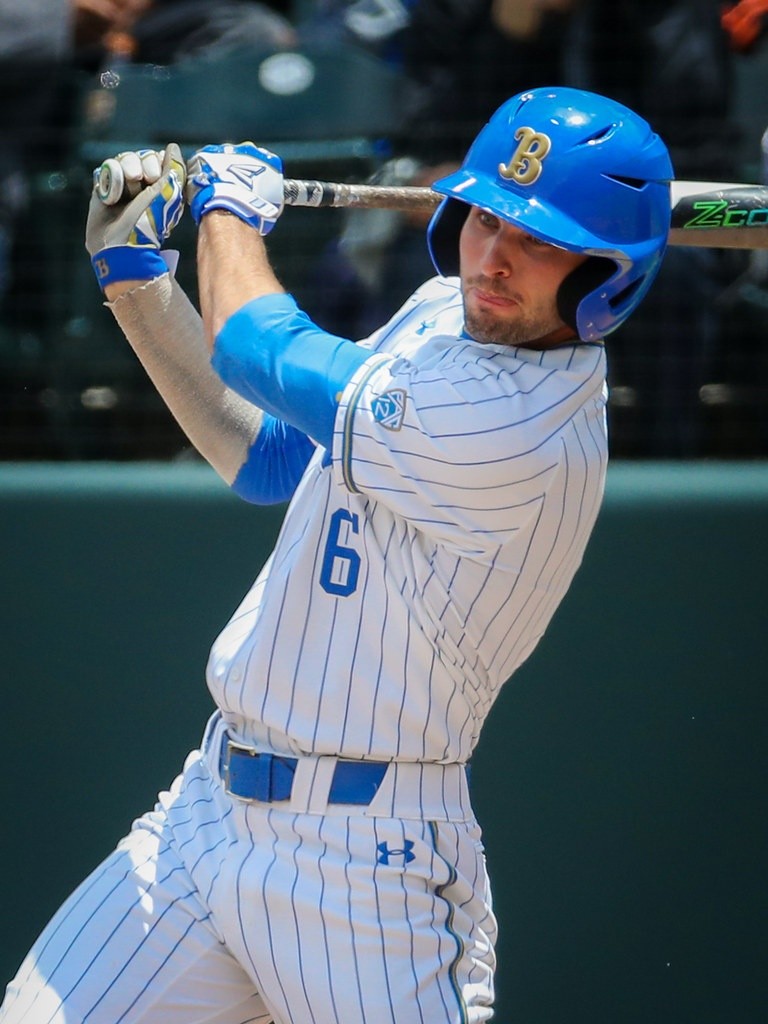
[204,713,470,803]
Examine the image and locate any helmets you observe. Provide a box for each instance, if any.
[425,85,675,343]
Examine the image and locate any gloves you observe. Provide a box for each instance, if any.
[83,142,187,288]
[187,141,284,236]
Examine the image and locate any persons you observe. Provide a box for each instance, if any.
[0,87,678,1024]
[0,0,768,460]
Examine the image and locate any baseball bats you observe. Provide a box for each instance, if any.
[97,155,768,249]
